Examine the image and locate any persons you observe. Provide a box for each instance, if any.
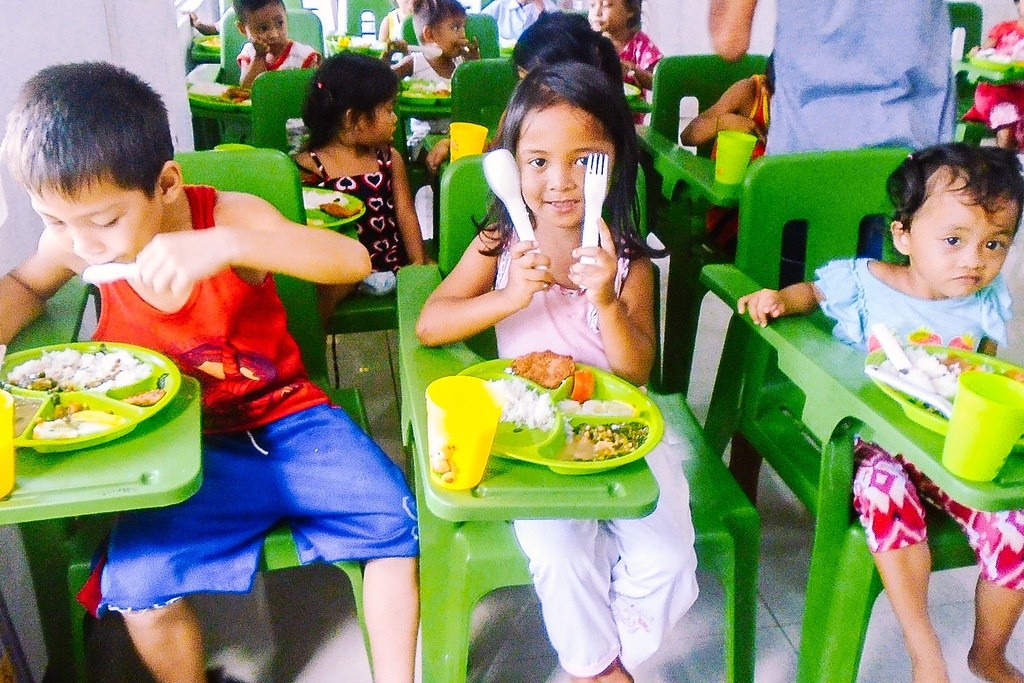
[233,0,321,88]
[961,0,1024,153]
[0,60,421,682]
[378,0,414,42]
[391,0,663,171]
[737,142,1024,683]
[187,5,234,36]
[708,0,958,156]
[415,63,699,683]
[291,53,436,331]
[680,52,775,259]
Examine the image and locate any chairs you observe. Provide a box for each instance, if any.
[0,1,1024,683]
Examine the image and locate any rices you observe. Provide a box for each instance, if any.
[187,80,228,97]
[879,346,962,401]
[6,347,152,396]
[302,189,349,208]
[485,367,560,432]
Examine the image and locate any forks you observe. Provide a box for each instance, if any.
[579,152,608,288]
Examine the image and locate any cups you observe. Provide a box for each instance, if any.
[715,130,757,185]
[425,375,502,491]
[449,122,488,162]
[0,390,14,498]
[943,370,1024,482]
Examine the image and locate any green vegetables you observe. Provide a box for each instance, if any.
[571,421,649,459]
[398,77,430,94]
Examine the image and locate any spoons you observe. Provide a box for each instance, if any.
[484,148,547,287]
[408,44,443,57]
[83,263,134,283]
[266,53,274,63]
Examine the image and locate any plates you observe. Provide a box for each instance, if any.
[458,358,664,476]
[865,344,1024,452]
[301,186,367,226]
[1,340,182,453]
[396,81,452,106]
[187,84,253,112]
[192,35,221,53]
[498,46,515,54]
[622,82,641,99]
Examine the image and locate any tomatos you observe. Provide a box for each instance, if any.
[571,367,594,402]
